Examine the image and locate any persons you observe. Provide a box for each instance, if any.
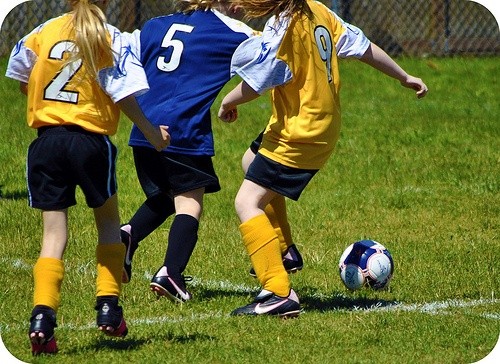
[116,0,264,302]
[218,0,429,318]
[5,0,171,356]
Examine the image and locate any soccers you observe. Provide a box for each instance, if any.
[339,239,394,292]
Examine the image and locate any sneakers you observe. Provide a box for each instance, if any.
[230,288,301,319]
[151,266,193,304]
[120,223,139,283]
[249,243,303,278]
[96,302,128,339]
[28,313,58,357]
